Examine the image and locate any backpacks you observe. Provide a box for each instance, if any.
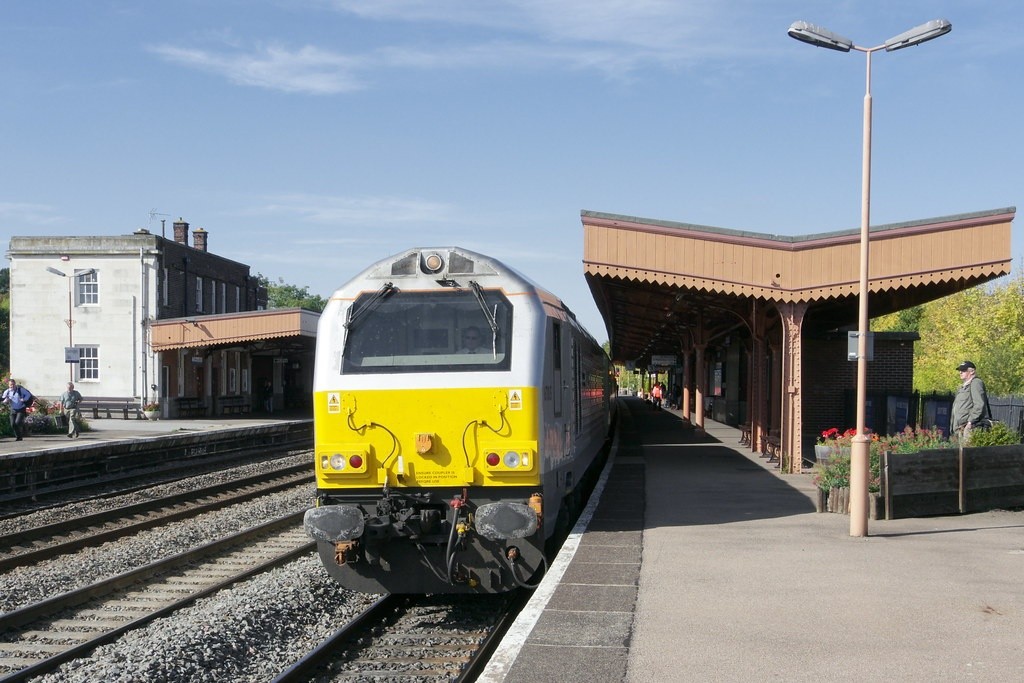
[18,386,33,407]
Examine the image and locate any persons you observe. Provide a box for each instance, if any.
[58,382,83,438]
[653,382,663,409]
[2,379,31,441]
[264,381,273,417]
[455,326,493,354]
[660,382,666,395]
[950,361,991,436]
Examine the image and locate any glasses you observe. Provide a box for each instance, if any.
[962,369,969,372]
[463,336,478,340]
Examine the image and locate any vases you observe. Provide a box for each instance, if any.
[144,410,162,420]
[814,445,851,459]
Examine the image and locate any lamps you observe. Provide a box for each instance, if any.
[676,293,687,300]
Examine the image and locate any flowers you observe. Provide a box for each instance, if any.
[817,426,879,446]
[143,401,161,411]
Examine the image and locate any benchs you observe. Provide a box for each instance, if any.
[78,397,134,420]
[177,397,209,418]
[218,395,250,416]
[738,424,752,447]
[760,434,780,468]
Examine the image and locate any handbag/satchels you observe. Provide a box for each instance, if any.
[972,418,1000,431]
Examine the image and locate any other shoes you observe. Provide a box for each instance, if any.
[76,433,79,438]
[16,438,22,441]
[67,434,73,438]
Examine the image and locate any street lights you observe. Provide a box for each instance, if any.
[46,265,95,383]
[787,18,954,540]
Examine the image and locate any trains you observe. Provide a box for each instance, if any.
[302,245,619,598]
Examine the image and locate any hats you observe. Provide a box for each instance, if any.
[956,361,975,370]
